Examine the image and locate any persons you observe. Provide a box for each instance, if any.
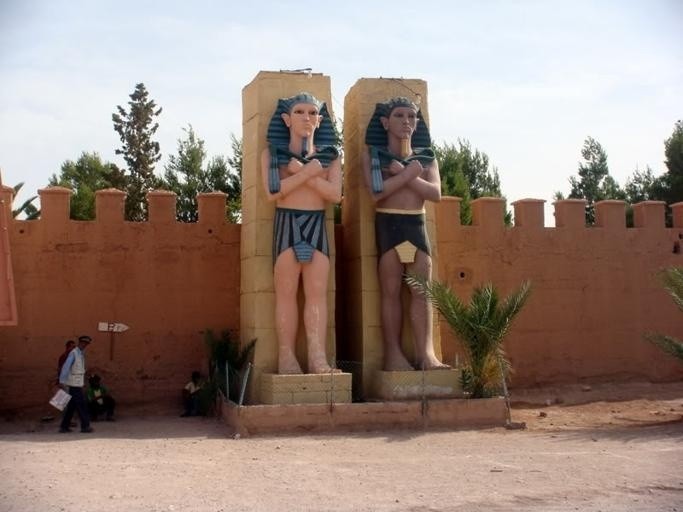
[180,371,205,416]
[263,93,341,375]
[48,336,115,433]
[364,97,451,371]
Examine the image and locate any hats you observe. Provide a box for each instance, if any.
[79,336,92,344]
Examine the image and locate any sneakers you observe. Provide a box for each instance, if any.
[59,426,72,433]
[80,427,96,433]
